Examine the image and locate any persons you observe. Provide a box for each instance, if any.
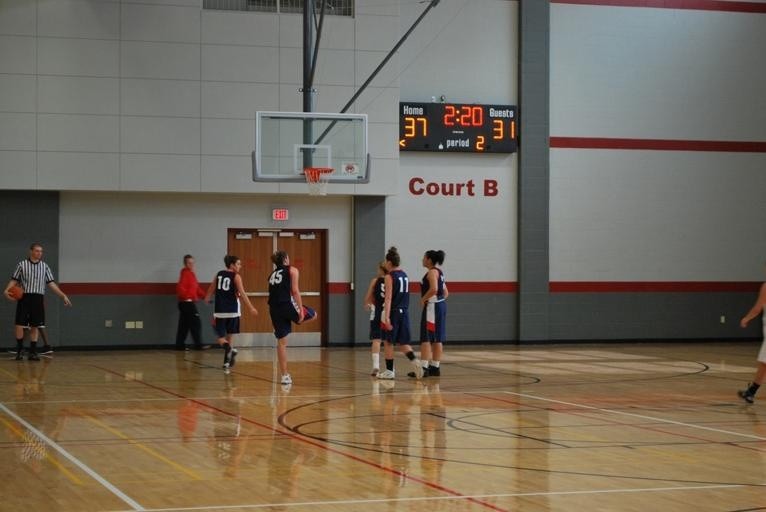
[204,255,258,369]
[7,294,54,354]
[204,375,449,510]
[267,250,318,384]
[376,247,424,379]
[362,258,389,375]
[174,254,212,351]
[406,249,449,378]
[4,242,73,361]
[736,283,766,404]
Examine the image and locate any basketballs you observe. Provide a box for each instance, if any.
[9,284,21,299]
[366,291,374,304]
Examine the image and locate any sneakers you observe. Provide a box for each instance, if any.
[376,369,395,380]
[369,366,380,376]
[223,348,239,369]
[39,346,54,355]
[280,373,293,384]
[411,360,425,380]
[429,365,440,377]
[408,366,429,377]
[28,354,40,361]
[16,352,23,360]
